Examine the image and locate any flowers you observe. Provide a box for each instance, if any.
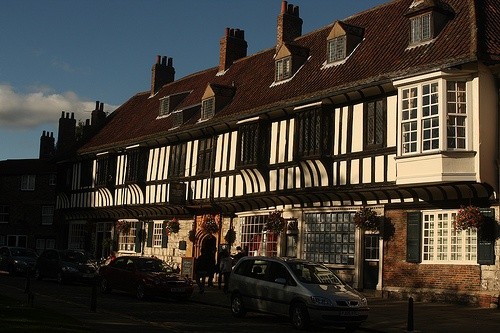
[201,216,219,234]
[115,220,132,235]
[456,201,483,231]
[165,217,181,236]
[354,208,379,229]
[263,211,286,233]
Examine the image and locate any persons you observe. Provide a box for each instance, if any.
[220,247,247,292]
[195,248,217,288]
[108,252,115,261]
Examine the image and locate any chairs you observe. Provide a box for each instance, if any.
[293,269,302,276]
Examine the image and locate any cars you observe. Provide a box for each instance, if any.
[34,249,98,285]
[98,255,194,301]
[0,246,40,276]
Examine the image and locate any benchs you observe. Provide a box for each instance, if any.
[246,266,266,278]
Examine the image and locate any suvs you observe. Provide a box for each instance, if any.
[227,256,371,331]
